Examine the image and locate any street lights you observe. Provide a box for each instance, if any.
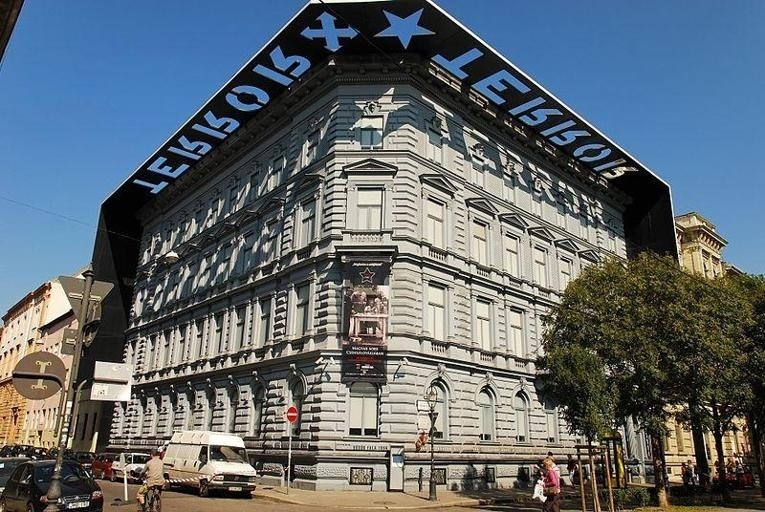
[426,382,437,504]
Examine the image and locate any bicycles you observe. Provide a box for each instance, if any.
[139,476,163,512]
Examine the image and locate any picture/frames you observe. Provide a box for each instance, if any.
[431,469,446,485]
[485,468,495,482]
[349,467,373,486]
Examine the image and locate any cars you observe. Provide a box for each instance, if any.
[0,459,105,512]
[0,443,160,492]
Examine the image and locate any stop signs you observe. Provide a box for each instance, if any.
[287,407,299,423]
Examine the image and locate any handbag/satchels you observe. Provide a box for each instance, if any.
[532,476,561,502]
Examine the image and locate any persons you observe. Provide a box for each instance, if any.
[688,459,698,485]
[712,456,756,488]
[681,461,691,485]
[136,448,164,509]
[567,453,578,486]
[537,451,561,512]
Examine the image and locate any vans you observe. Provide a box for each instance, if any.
[161,429,257,497]
